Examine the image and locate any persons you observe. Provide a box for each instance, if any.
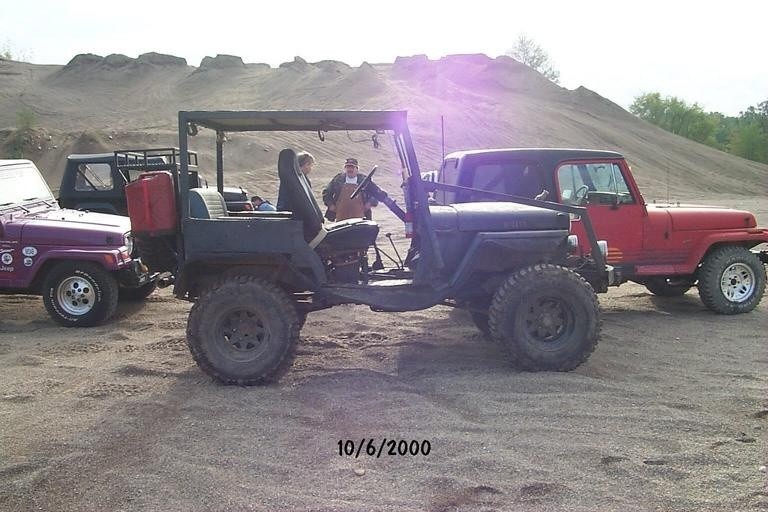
[319,158,380,223]
[250,195,278,213]
[296,150,315,189]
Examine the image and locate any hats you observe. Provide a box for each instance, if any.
[344,158,358,168]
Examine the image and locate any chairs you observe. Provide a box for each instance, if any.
[188,187,230,220]
[278,148,380,280]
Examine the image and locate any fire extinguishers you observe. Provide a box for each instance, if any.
[404,206,415,238]
